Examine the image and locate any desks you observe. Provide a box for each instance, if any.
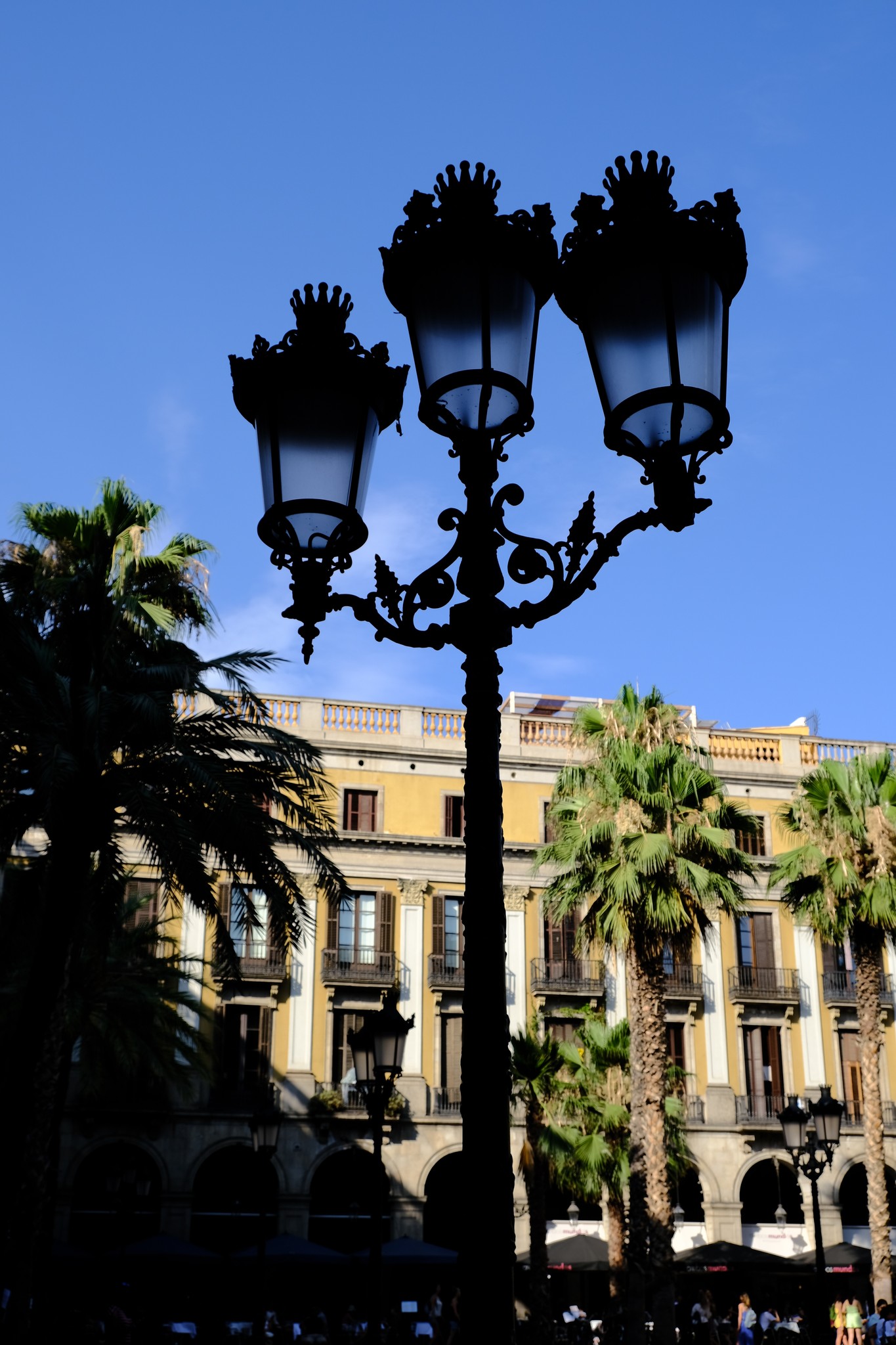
[775,1322,800,1345]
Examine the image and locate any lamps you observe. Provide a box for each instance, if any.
[672,1175,685,1222]
[567,1192,579,1220]
[771,1156,787,1223]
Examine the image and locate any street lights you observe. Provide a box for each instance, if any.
[345,983,415,1345]
[226,150,748,1345]
[776,1083,845,1345]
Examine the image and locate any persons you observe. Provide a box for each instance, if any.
[671,1287,804,1345]
[197,1283,635,1345]
[828,1289,896,1345]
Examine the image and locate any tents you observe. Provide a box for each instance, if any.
[117,1237,216,1259]
[672,1241,792,1272]
[517,1235,610,1272]
[351,1235,457,1264]
[234,1231,348,1266]
[788,1241,896,1277]
